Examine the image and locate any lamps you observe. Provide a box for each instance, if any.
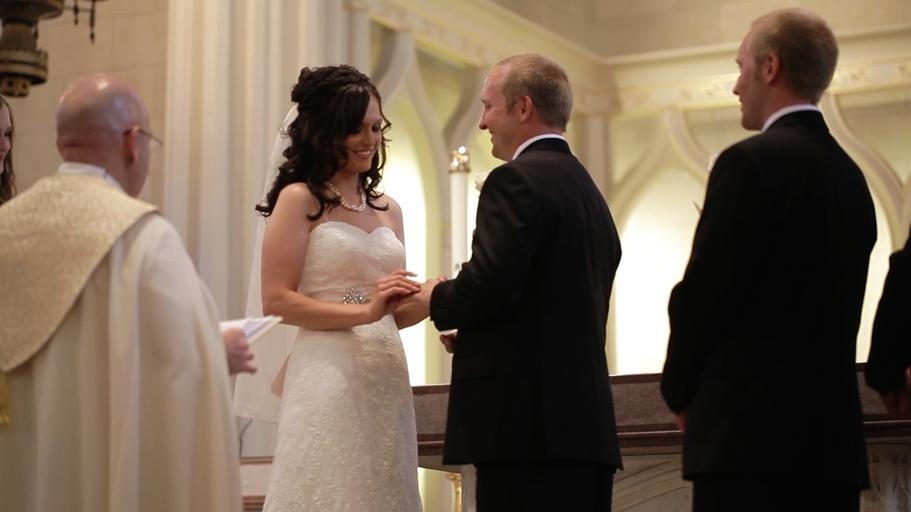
[0,0,67,97]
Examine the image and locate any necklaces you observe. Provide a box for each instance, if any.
[326,180,369,214]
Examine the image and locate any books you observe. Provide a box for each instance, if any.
[220,312,285,345]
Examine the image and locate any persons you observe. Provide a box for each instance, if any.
[399,52,628,509]
[1,96,21,214]
[863,227,911,428]
[242,60,454,510]
[1,71,260,510]
[656,5,876,511]
